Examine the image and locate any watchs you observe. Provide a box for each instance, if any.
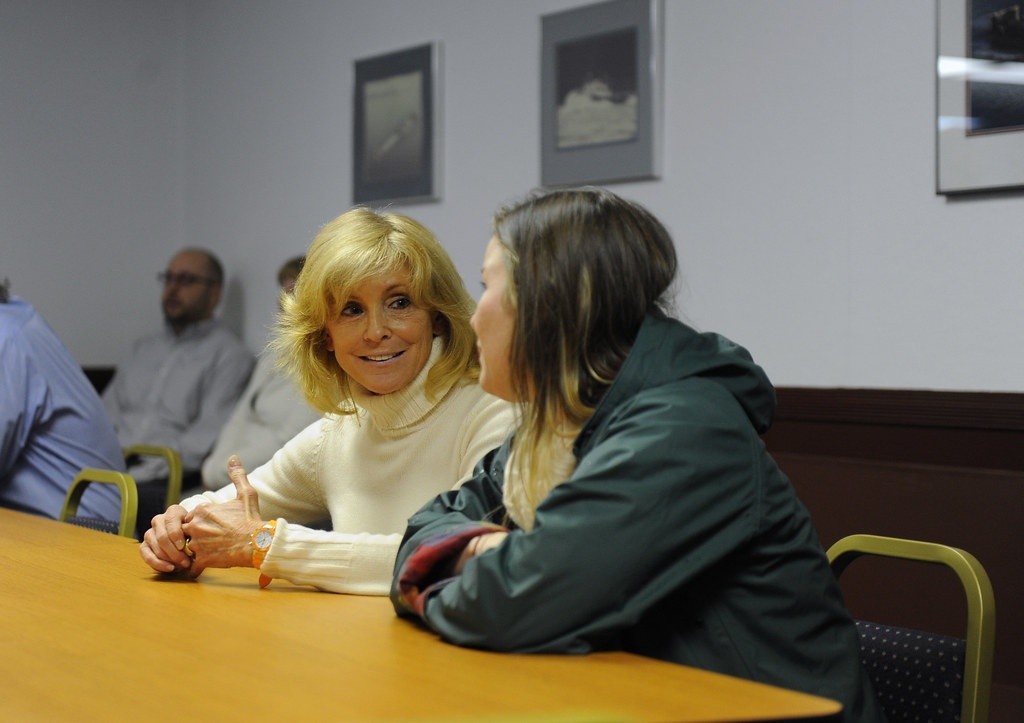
[251,520,276,588]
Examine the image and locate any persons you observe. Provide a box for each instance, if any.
[392,191,878,723]
[187,255,324,496]
[0,280,127,520]
[140,207,523,597]
[99,247,252,483]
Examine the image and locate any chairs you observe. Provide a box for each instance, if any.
[122,441,185,527]
[60,465,140,541]
[828,531,996,723]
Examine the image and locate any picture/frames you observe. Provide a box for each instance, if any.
[932,0,1024,193]
[538,1,665,190]
[348,36,448,207]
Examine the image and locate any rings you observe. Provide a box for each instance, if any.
[184,539,193,556]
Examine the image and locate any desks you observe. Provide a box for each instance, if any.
[0,505,844,722]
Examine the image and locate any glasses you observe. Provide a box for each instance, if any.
[157,271,212,287]
